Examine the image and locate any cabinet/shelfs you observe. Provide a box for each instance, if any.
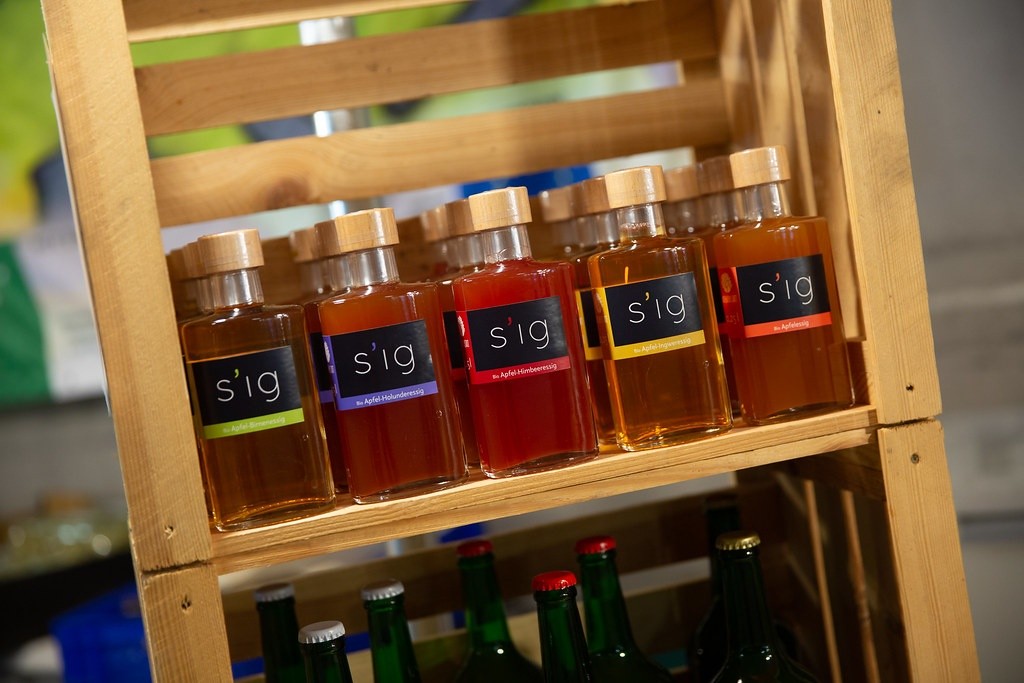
[35,1,981,683]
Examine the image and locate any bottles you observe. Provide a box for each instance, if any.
[171,147,853,683]
